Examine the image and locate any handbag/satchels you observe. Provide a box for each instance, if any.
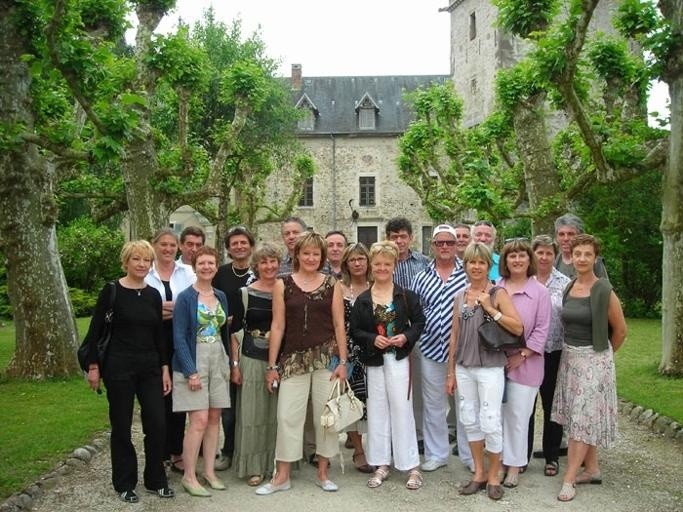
[76,314,112,374]
[474,318,529,352]
[320,376,368,436]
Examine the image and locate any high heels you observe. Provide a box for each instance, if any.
[201,469,227,491]
[460,472,488,495]
[179,478,213,498]
[488,472,504,499]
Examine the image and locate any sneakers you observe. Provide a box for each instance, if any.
[146,487,176,499]
[313,475,339,492]
[245,475,264,488]
[254,478,294,495]
[116,487,139,503]
[574,469,605,486]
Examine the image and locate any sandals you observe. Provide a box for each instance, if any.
[502,468,519,488]
[169,434,568,476]
[367,468,392,488]
[405,470,425,490]
[556,479,577,502]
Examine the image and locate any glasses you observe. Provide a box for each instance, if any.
[433,240,458,247]
[227,225,248,234]
[473,220,493,228]
[504,236,529,244]
[371,244,399,254]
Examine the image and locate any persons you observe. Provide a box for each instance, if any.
[78,214,628,502]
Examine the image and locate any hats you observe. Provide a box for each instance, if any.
[432,223,459,240]
